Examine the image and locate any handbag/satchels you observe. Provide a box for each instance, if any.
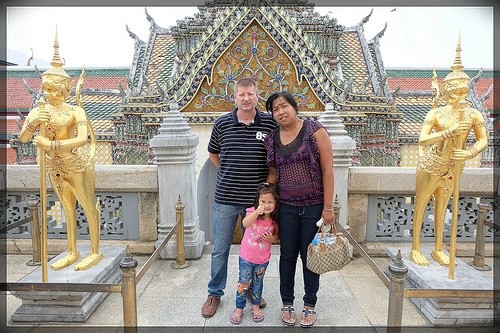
[305,221,354,275]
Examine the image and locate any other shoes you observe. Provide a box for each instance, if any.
[202,296,221,318]
[247,290,266,308]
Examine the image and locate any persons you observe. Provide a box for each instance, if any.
[19,72,104,271]
[409,71,487,267]
[263,91,352,327]
[230,181,278,324]
[201,78,281,318]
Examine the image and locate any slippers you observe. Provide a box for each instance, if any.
[280,306,296,326]
[299,309,318,327]
[251,308,265,322]
[229,309,244,323]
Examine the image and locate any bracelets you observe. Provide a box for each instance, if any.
[323,206,333,211]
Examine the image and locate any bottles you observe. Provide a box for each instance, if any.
[312,234,338,245]
